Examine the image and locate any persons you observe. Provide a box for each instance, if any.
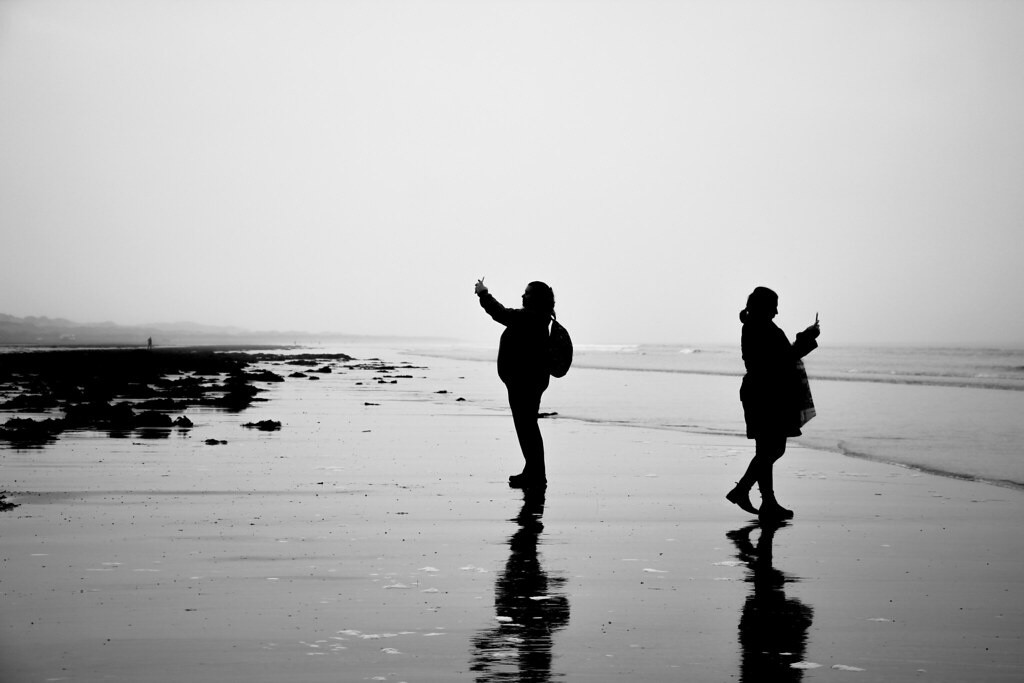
[148,337,152,349]
[474,277,574,486]
[726,286,821,518]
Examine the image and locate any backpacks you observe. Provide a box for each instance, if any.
[547,318,574,379]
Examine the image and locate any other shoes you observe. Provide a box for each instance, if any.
[758,495,794,518]
[725,482,759,515]
[508,472,547,484]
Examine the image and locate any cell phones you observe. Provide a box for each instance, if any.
[816,313,818,322]
[481,277,484,283]
